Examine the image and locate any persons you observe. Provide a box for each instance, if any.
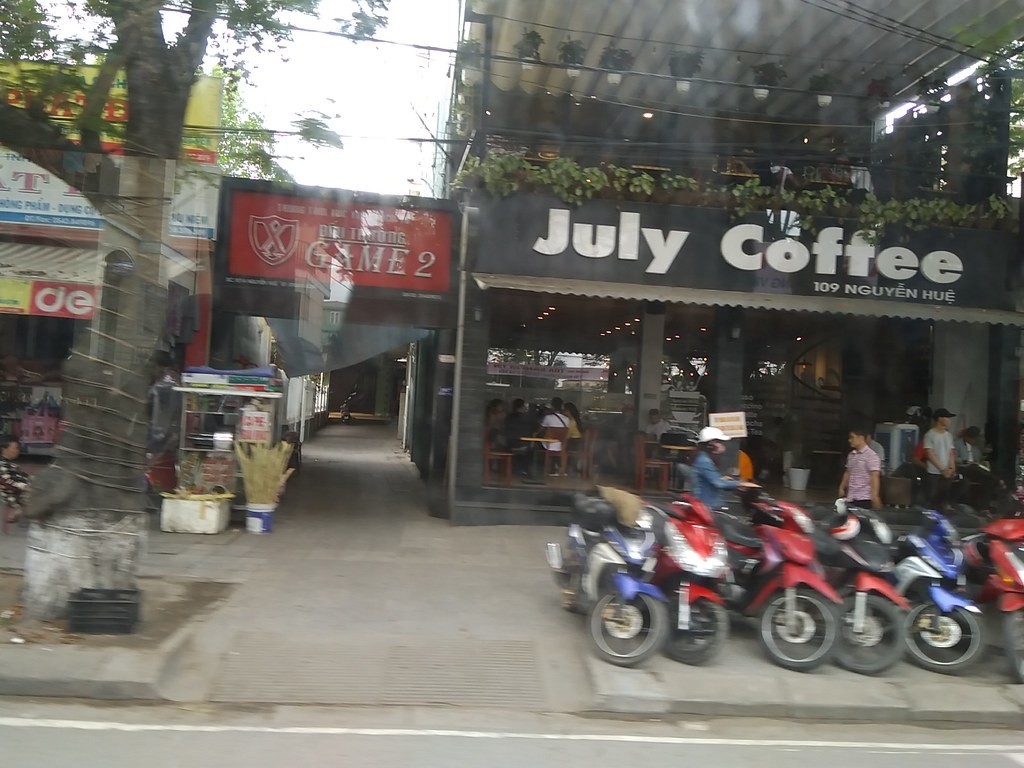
[838,427,882,510]
[735,145,775,187]
[646,408,670,459]
[862,424,885,476]
[484,396,583,479]
[953,426,1000,513]
[674,462,692,490]
[912,441,928,467]
[688,426,763,509]
[0,436,35,508]
[606,405,635,472]
[922,409,956,495]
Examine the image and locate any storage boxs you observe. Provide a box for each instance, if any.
[66,585,138,635]
[160,499,231,534]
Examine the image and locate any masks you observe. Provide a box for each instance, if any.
[708,442,728,454]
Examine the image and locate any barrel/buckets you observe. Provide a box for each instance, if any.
[787,468,810,491]
[246,503,277,533]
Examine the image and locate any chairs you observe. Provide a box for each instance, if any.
[564,428,598,475]
[481,426,513,479]
[530,429,569,477]
[633,432,670,491]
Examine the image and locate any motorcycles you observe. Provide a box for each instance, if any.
[961,519,1024,683]
[890,506,986,676]
[807,491,918,676]
[646,488,733,665]
[718,483,847,665]
[545,483,681,668]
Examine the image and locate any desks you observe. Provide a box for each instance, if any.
[722,171,759,184]
[521,436,560,485]
[634,165,672,178]
[806,179,847,191]
[524,156,553,168]
[661,445,696,493]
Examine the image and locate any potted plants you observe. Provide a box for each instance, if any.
[561,37,588,77]
[514,29,543,71]
[810,74,843,106]
[753,63,787,100]
[598,42,634,86]
[919,77,948,113]
[869,77,896,107]
[445,39,482,141]
[668,50,703,93]
[451,153,1022,245]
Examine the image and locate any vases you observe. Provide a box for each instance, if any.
[791,467,811,491]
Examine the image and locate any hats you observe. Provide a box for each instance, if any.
[967,427,981,438]
[933,407,958,418]
[697,426,732,444]
